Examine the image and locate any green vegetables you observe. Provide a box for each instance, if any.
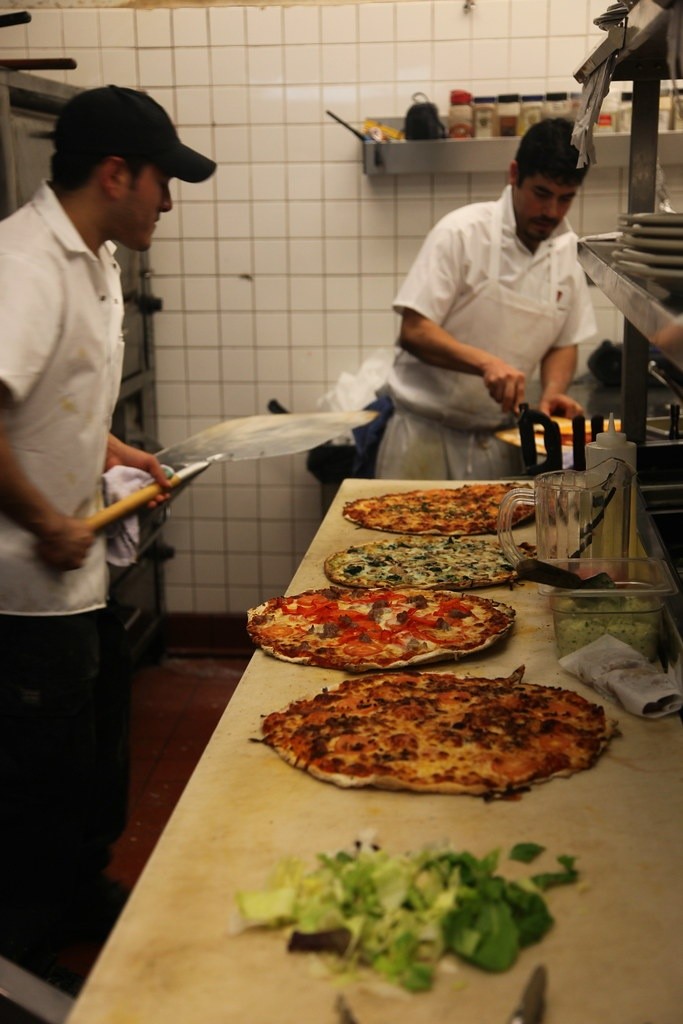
[234,841,580,993]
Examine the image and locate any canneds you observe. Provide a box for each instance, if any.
[472,88,683,138]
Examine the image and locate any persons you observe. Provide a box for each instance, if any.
[375,115,597,482]
[0,81,219,1000]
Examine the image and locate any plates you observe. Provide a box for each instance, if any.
[609,211,683,277]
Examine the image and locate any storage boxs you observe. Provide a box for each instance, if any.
[539,558,679,662]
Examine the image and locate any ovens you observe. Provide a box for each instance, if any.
[0,67,177,705]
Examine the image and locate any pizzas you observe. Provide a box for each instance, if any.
[325,535,539,589]
[248,662,618,798]
[342,482,535,534]
[246,584,518,672]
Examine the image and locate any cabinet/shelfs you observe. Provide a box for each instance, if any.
[571,1,681,661]
[1,69,166,669]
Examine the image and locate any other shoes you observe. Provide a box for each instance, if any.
[40,963,83,996]
[83,887,125,945]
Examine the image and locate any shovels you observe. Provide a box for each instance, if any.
[515,555,616,608]
[82,408,381,531]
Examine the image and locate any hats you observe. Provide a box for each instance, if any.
[51,84,217,183]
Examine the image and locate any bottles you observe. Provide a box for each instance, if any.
[447,88,633,138]
[585,412,638,559]
[658,87,683,133]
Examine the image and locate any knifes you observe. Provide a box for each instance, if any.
[512,558,627,610]
[506,963,549,1024]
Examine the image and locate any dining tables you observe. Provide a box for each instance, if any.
[67,478,683,1024]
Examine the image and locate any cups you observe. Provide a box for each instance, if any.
[496,456,638,585]
